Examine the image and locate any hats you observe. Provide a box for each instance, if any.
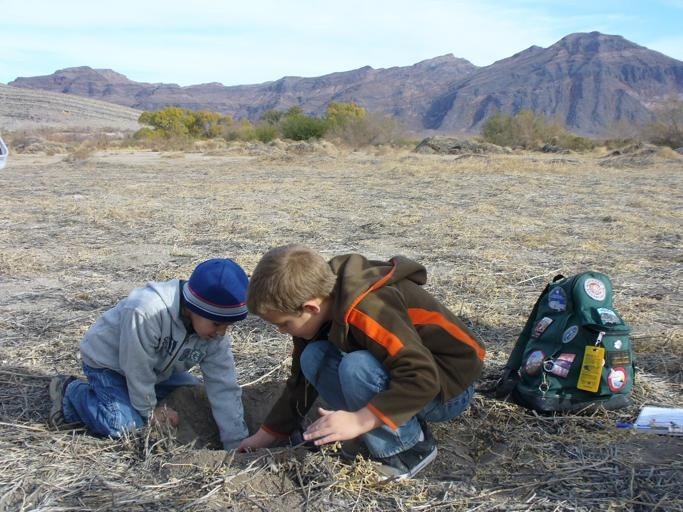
[181,258,249,323]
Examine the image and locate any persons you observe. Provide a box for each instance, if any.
[42,251,257,453]
[236,241,488,484]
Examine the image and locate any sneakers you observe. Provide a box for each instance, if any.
[49,376,78,427]
[375,415,439,488]
[289,431,368,455]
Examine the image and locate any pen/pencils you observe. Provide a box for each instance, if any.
[617,424,651,429]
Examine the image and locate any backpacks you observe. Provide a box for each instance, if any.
[493,271,635,415]
